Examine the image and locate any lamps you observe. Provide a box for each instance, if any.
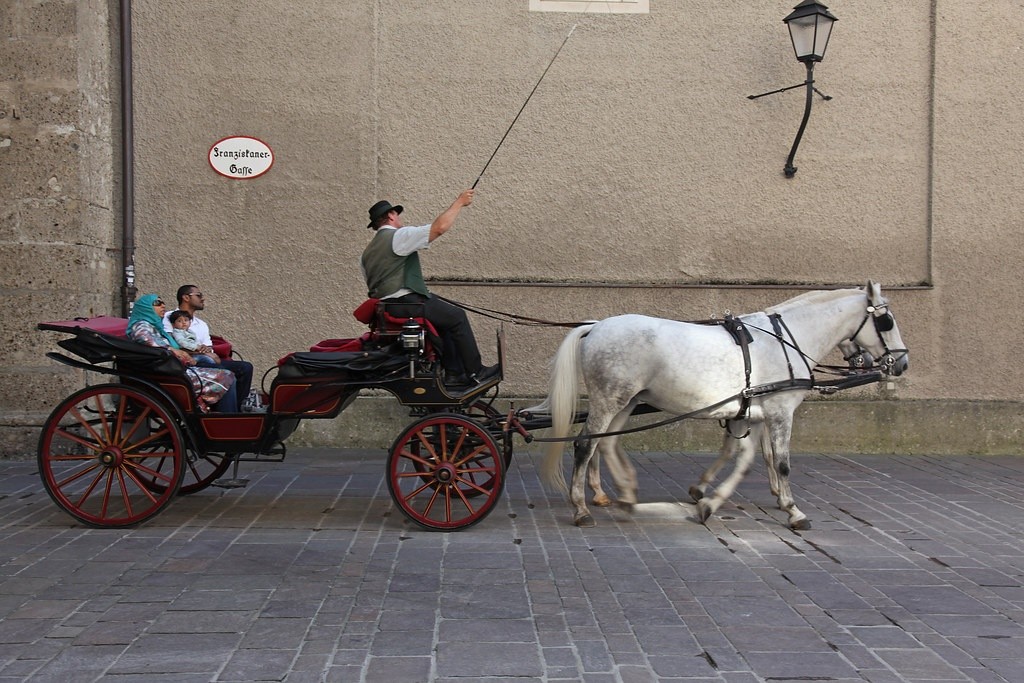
[746,0,840,175]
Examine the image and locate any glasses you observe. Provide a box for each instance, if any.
[152,300,165,306]
[188,292,202,299]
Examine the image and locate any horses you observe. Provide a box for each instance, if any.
[514,278,909,529]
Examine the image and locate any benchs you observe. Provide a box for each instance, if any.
[94,323,244,399]
[274,332,371,378]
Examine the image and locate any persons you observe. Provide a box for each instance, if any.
[126,294,236,413]
[361,189,500,385]
[163,285,253,409]
[170,310,221,365]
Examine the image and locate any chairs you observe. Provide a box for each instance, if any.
[370,300,442,359]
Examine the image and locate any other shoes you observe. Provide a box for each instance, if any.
[197,396,209,414]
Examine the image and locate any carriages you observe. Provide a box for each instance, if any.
[42,278,911,533]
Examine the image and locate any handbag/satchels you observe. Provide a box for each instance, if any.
[267,347,411,418]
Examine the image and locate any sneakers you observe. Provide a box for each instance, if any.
[444,372,478,386]
[469,363,498,387]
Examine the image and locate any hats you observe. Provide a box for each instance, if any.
[366,201,403,228]
